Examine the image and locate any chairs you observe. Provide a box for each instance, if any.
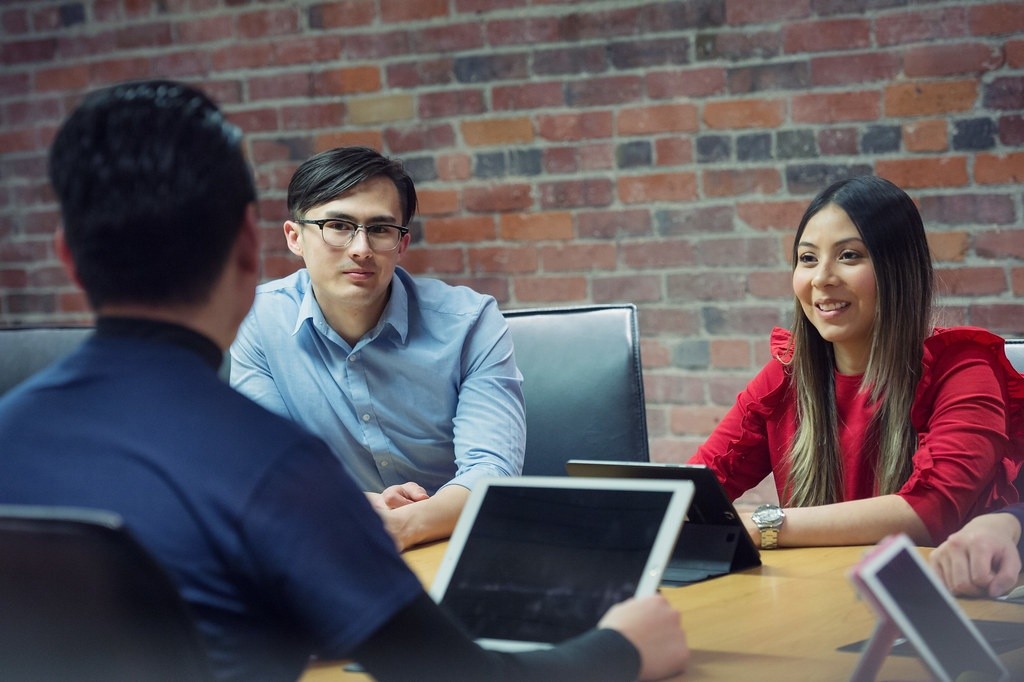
[501,302,650,475]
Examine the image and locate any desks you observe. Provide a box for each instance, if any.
[299,539,1024,682]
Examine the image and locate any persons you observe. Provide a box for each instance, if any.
[929,504,1024,599]
[685,175,1024,550]
[1,80,689,682]
[229,146,527,554]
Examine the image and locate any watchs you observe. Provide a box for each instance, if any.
[751,504,786,550]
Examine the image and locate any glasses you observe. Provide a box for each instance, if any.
[294,218,409,252]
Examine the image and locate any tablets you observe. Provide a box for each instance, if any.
[860,529,1009,682]
[567,460,760,569]
[431,478,694,654]
